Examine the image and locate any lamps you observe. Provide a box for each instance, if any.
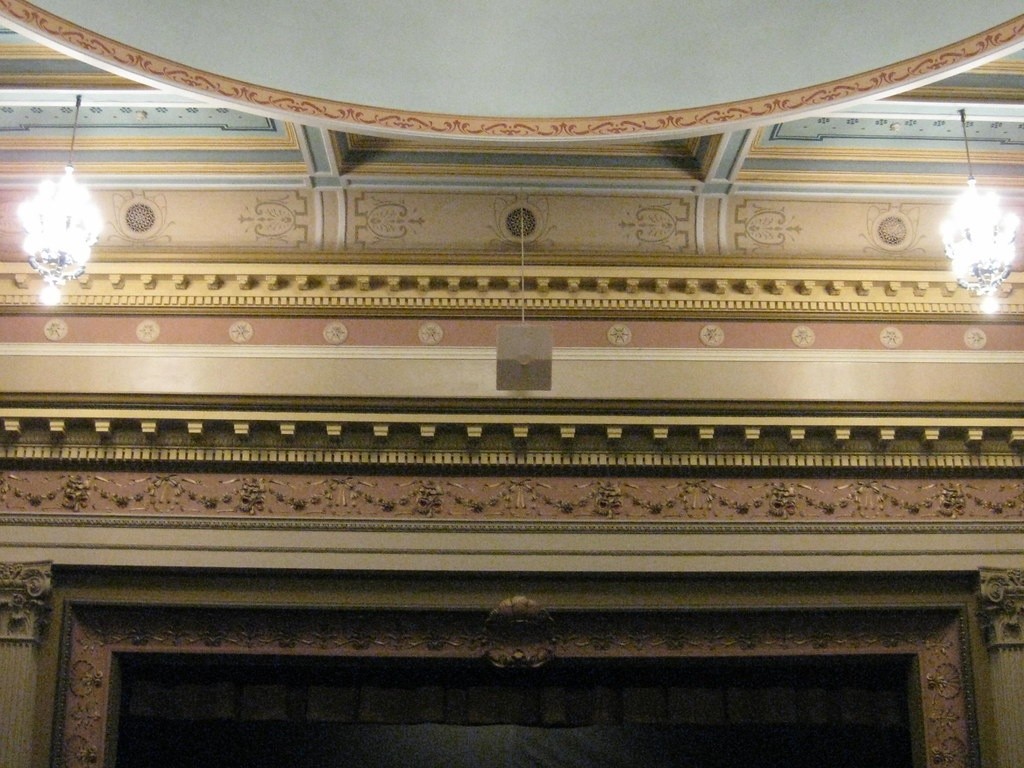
[940,108,1019,315]
[496,184,553,390]
[17,95,103,305]
